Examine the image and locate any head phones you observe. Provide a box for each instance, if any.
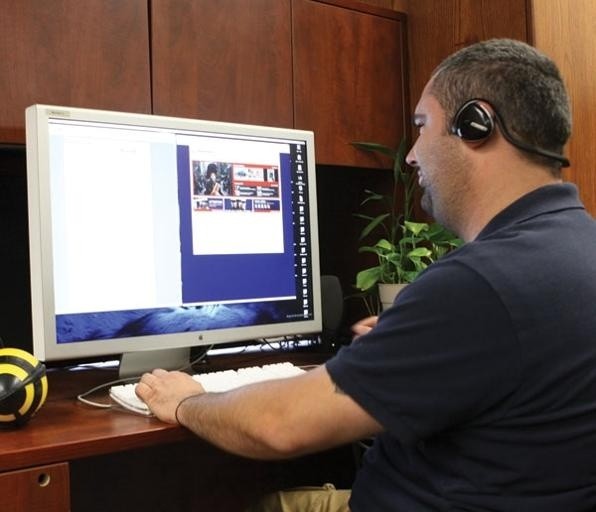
[0,348,48,426]
[450,98,570,167]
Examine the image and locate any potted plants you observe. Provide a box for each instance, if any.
[346,133,465,312]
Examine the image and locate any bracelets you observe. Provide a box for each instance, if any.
[175,392,206,427]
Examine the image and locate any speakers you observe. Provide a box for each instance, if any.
[320,275,344,353]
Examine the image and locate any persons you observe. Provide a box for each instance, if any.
[135,38,596,512]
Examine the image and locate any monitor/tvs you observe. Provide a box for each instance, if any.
[24,103,322,384]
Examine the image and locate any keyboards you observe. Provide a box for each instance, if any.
[108,361,308,416]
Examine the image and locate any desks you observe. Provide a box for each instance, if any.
[0,340,357,512]
